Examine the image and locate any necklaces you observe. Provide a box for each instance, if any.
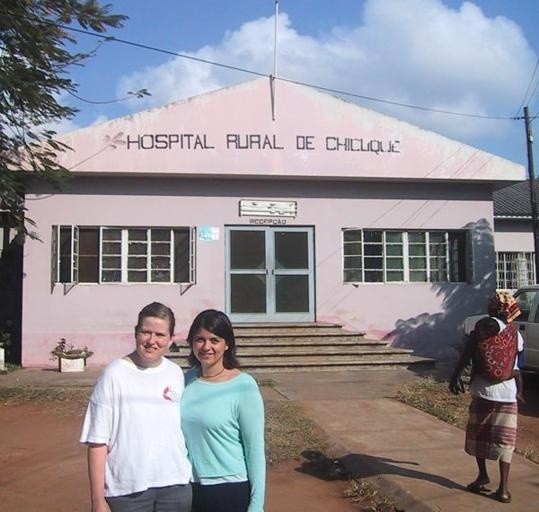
[200,365,224,377]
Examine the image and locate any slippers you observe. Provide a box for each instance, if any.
[467,481,491,492]
[496,489,511,503]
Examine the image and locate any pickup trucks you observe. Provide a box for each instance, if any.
[463,285,539,376]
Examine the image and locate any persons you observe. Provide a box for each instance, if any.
[472,316,524,404]
[180,310,266,512]
[79,301,194,511]
[449,292,525,502]
